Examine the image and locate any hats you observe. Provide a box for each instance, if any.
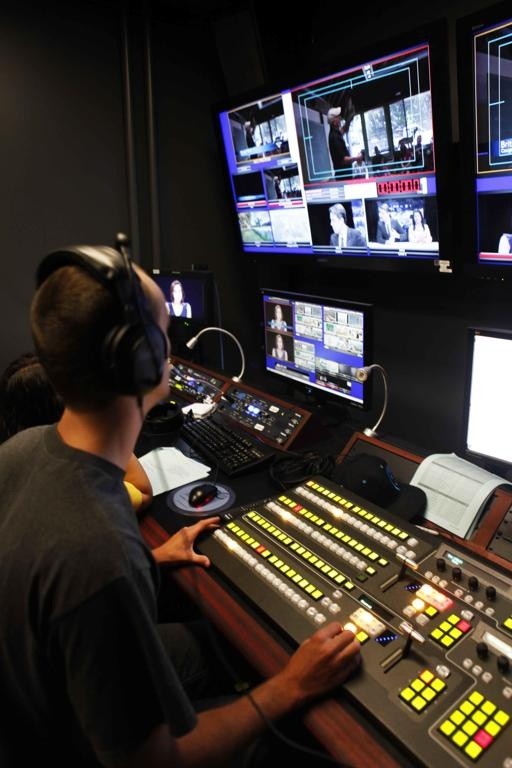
[244,121,251,129]
[327,106,341,123]
[274,176,278,181]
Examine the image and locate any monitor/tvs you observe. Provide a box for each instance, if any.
[219,41,461,275]
[146,268,207,336]
[260,288,374,412]
[462,324,512,475]
[465,15,512,288]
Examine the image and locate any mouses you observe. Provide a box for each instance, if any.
[188,484,218,508]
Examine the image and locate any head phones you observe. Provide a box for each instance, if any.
[33,232,172,397]
[267,449,335,492]
[142,401,185,434]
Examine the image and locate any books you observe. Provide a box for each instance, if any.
[136,445,211,498]
[410,451,512,540]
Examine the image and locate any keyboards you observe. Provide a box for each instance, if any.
[182,412,276,480]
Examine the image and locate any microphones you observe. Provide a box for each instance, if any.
[185,327,246,384]
[356,364,389,438]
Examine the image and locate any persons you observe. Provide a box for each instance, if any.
[375,204,407,243]
[164,280,192,318]
[326,107,362,180]
[1,241,363,768]
[272,335,288,362]
[0,362,154,512]
[408,210,432,243]
[329,205,366,247]
[271,305,288,331]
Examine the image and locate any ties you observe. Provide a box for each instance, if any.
[340,237,345,246]
